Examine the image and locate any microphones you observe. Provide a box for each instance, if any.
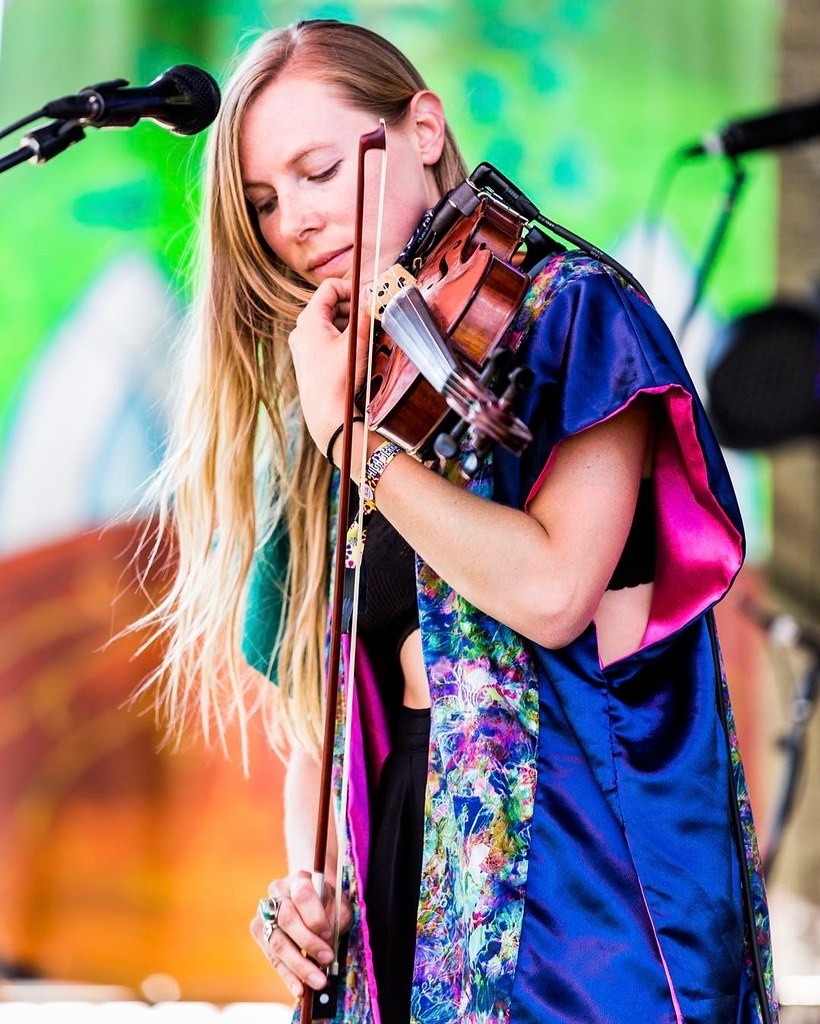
[41,64,221,136]
[702,97,820,157]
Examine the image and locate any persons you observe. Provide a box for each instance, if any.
[162,19,777,1023]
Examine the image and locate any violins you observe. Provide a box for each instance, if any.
[349,161,540,461]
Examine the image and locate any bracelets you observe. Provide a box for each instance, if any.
[327,417,366,466]
[344,442,402,569]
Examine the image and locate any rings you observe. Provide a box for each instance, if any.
[260,896,282,922]
[263,920,278,943]
